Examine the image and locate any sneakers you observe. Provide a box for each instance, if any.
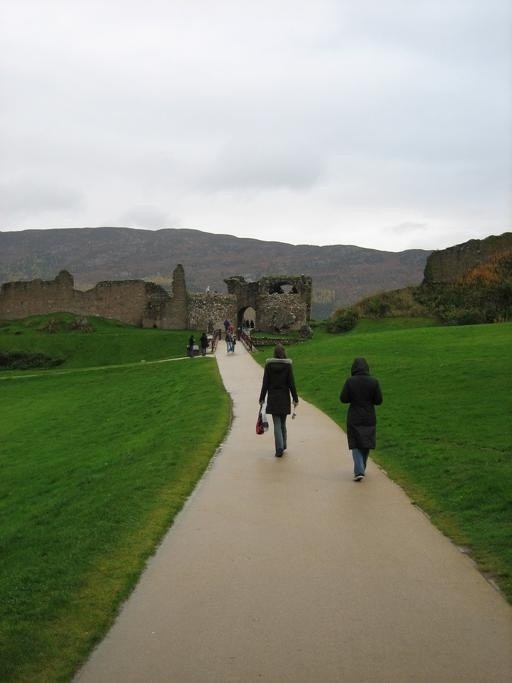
[275,447,288,456]
[353,467,366,481]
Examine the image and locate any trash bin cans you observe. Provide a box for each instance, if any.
[187,345,199,356]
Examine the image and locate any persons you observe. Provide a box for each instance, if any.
[246,320,254,328]
[259,344,298,457]
[224,318,236,353]
[340,358,382,481]
[189,333,209,358]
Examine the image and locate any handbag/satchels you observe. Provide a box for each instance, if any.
[256,402,264,435]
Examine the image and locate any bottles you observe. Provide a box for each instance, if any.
[262,414,269,431]
[292,408,297,420]
